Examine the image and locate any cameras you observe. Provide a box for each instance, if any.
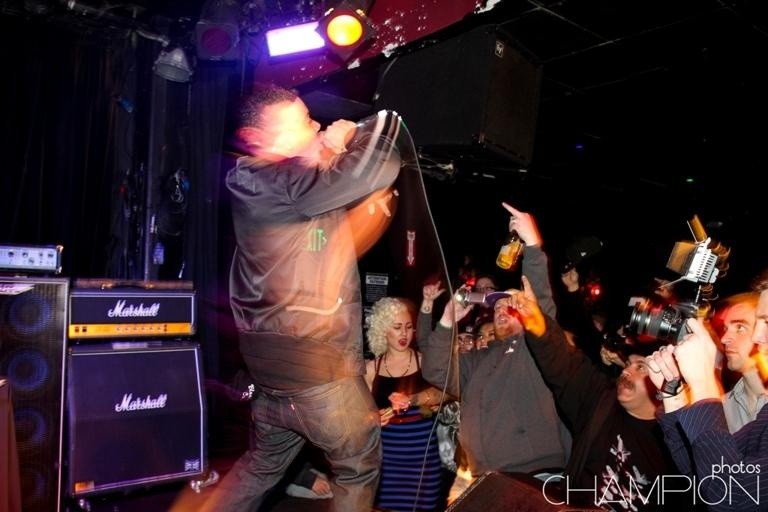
[457,289,486,307]
[629,298,699,396]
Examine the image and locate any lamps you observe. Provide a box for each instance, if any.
[315,0,378,63]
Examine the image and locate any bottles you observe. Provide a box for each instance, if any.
[496,228,522,270]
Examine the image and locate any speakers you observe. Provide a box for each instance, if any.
[0,277,69,512]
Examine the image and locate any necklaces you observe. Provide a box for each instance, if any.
[381,349,413,379]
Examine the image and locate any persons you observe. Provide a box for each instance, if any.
[416,200,768,512]
[195,81,413,511]
[361,294,443,511]
[290,466,332,496]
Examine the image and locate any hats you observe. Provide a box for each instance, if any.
[616,342,665,369]
[484,287,521,311]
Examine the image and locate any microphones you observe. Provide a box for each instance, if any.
[356,109,387,132]
